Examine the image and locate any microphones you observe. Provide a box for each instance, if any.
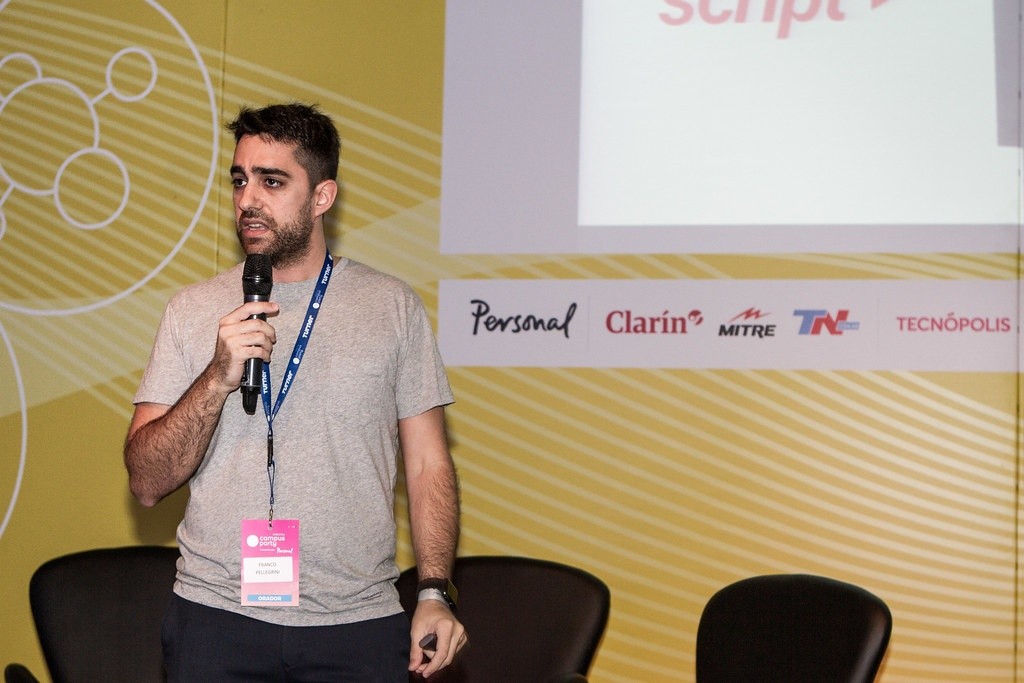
[239,253,273,416]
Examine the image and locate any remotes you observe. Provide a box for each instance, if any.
[419,633,437,652]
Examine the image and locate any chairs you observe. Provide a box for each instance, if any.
[696,574,892,683]
[3,545,184,683]
[395,555,611,683]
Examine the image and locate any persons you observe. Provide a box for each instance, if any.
[125,102,470,683]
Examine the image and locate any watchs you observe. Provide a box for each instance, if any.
[416,578,459,612]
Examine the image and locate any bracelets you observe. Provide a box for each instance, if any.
[418,589,449,607]
